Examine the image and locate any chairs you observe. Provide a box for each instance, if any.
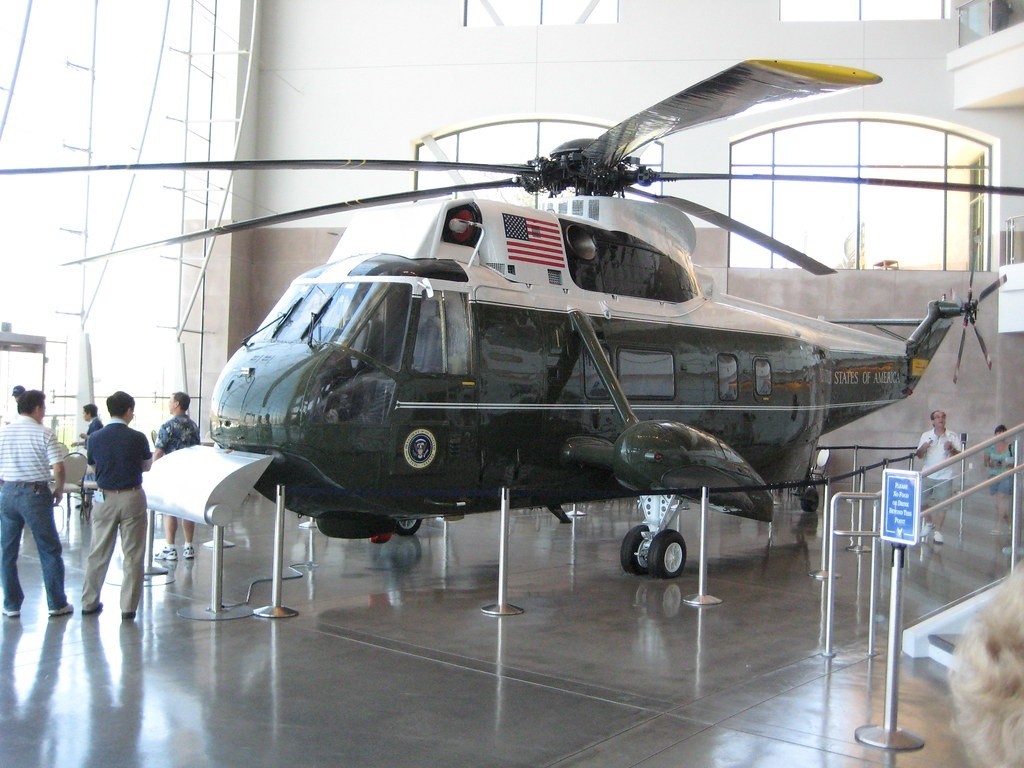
[48,452,89,520]
[80,463,98,516]
[411,314,441,373]
[58,441,70,457]
[349,318,375,370]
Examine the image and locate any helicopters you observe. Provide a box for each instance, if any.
[0,56,1022,587]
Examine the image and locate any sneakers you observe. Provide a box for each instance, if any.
[48,603,73,614]
[153,547,178,560]
[182,546,195,558]
[2,607,19,616]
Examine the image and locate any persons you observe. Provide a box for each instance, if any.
[76,405,104,509]
[81,391,152,619]
[0,390,73,616]
[916,410,963,543]
[2,385,37,427]
[949,569,1024,768]
[152,392,201,560]
[984,426,1015,535]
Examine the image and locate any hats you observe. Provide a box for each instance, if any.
[12,386,26,396]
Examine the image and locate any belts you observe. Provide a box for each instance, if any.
[98,488,128,492]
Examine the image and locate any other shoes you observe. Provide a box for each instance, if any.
[122,611,136,619]
[82,602,103,614]
[75,504,93,509]
[921,523,935,536]
[934,530,944,543]
